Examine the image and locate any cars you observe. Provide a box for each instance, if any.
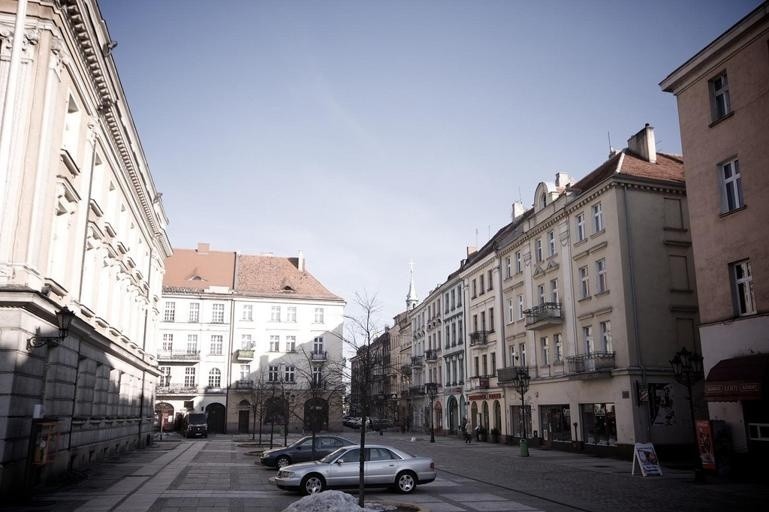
[258,435,359,471]
[272,443,434,496]
[342,415,388,430]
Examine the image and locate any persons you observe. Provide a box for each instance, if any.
[465,420,473,443]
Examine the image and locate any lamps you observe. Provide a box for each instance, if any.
[26,303,75,350]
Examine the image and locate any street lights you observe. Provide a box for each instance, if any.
[668,345,708,482]
[422,383,438,442]
[391,394,397,427]
[508,367,531,457]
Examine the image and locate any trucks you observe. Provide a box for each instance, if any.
[180,409,209,438]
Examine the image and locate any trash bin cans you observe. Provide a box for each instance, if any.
[520,439,529,457]
